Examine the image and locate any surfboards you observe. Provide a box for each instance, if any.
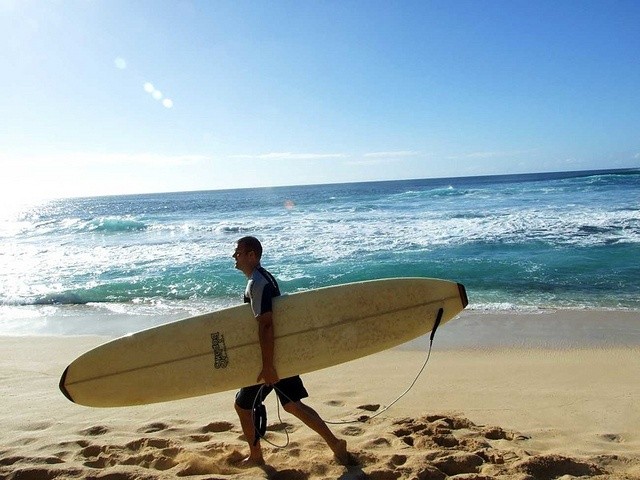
[58,277,470,408]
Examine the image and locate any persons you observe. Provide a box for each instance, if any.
[232,236,347,467]
